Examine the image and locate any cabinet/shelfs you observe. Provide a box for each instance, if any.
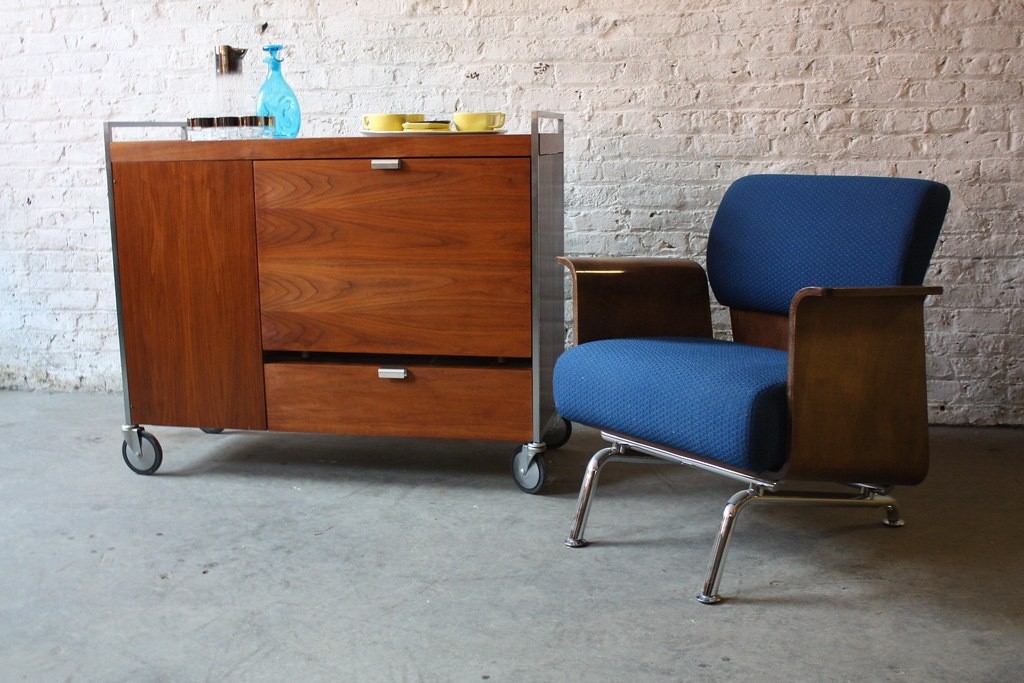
[101,109,574,496]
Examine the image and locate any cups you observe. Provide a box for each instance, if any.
[187,117,215,141]
[216,116,239,140]
[263,116,275,139]
[364,115,404,131]
[454,113,504,131]
[399,113,424,123]
[240,116,263,138]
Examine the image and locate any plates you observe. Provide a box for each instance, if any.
[403,122,451,130]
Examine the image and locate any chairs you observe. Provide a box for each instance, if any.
[554,173,951,606]
